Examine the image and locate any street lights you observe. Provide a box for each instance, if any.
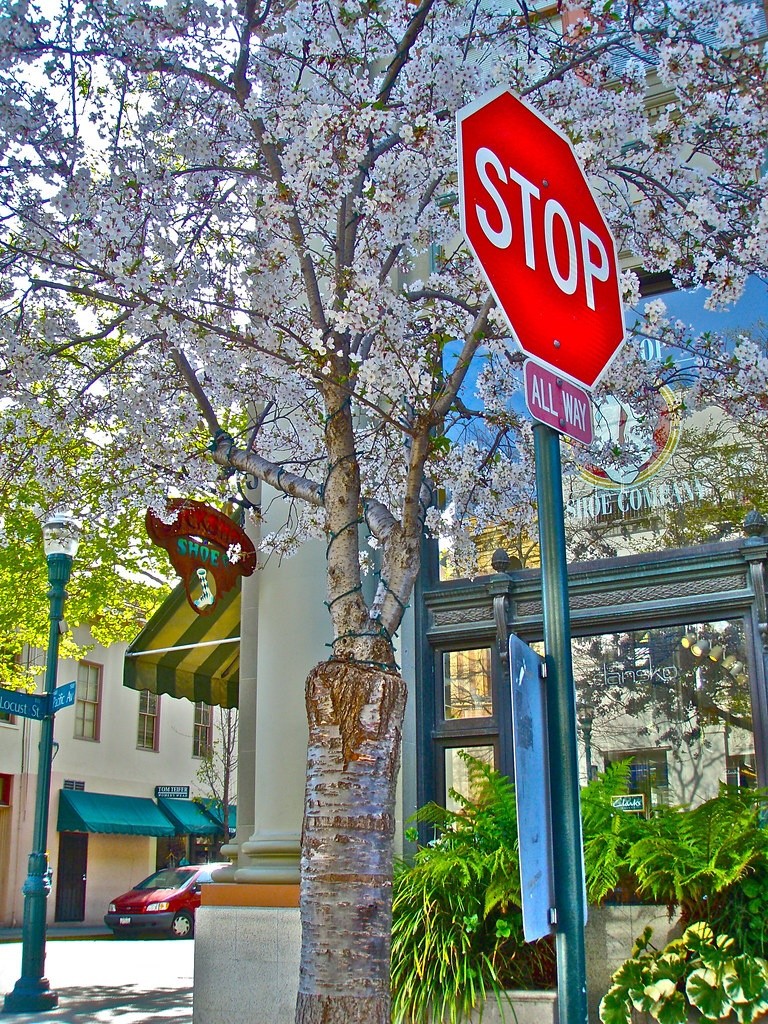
[4,509,85,1012]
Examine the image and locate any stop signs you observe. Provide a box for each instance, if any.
[457,82,629,390]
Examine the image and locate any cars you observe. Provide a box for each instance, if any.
[104,861,232,939]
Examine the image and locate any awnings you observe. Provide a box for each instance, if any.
[56,788,176,837]
[196,797,236,837]
[157,795,219,836]
[125,570,242,711]
